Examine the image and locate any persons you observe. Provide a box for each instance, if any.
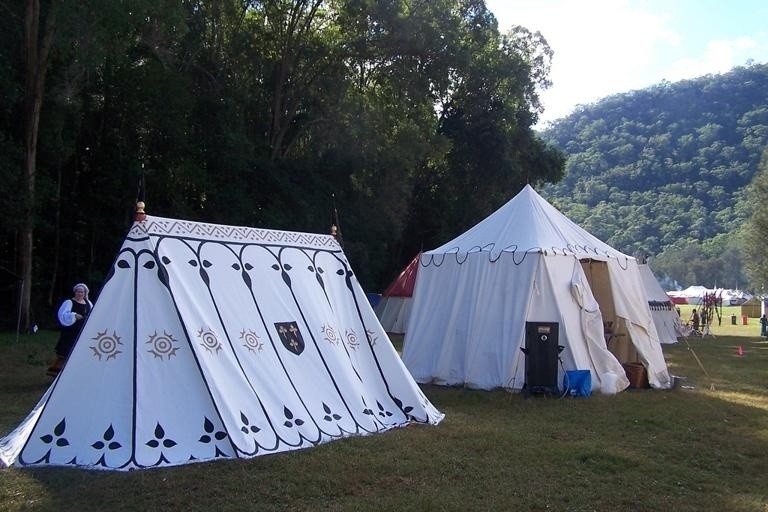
[759,314,767,336]
[691,309,699,330]
[56,283,93,357]
[700,308,707,332]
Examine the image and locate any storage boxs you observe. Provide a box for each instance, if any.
[621,362,648,389]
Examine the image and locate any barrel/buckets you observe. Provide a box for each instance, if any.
[732,316,737,323]
[565,370,592,397]
[742,316,748,324]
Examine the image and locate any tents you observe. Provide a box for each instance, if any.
[636,263,685,345]
[0,200,442,473]
[665,283,753,306]
[367,180,675,399]
[372,250,424,335]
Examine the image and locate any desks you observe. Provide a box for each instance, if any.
[603,333,625,349]
